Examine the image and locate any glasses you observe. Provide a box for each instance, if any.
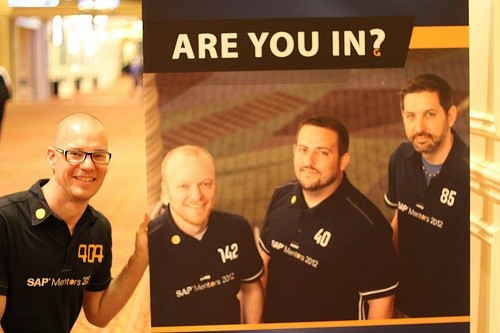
[53,146,111,164]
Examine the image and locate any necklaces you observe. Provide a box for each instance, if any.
[423,166,440,177]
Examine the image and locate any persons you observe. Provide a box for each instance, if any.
[0,113,149,333]
[148,145,265,327]
[384,72,470,319]
[258,117,399,323]
